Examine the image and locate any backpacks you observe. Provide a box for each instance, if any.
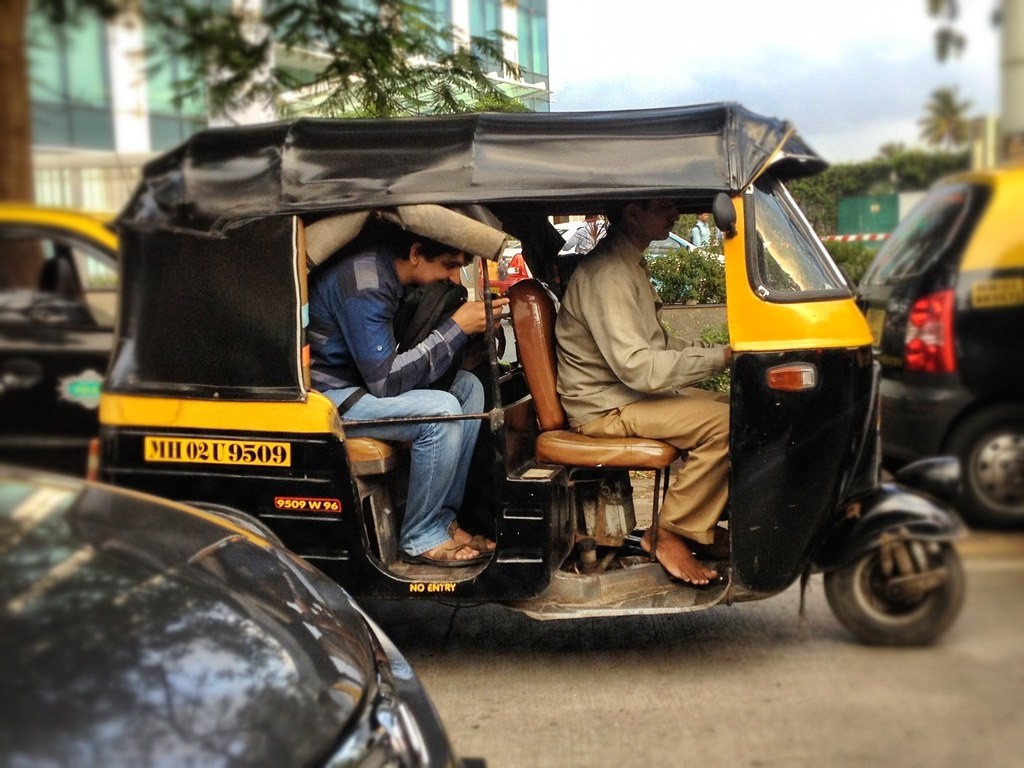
[394,279,468,392]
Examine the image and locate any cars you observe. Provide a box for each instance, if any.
[553,218,607,255]
[851,166,1024,533]
[644,231,726,272]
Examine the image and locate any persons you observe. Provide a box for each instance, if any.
[305,221,510,566]
[553,201,732,585]
[692,213,711,249]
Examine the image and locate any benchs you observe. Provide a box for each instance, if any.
[339,432,424,566]
[509,271,674,563]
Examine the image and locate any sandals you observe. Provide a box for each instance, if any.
[448,520,496,557]
[398,540,485,566]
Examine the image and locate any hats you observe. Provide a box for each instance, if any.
[585,214,599,221]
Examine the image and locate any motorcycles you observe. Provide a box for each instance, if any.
[0,203,125,478]
[85,98,968,654]
